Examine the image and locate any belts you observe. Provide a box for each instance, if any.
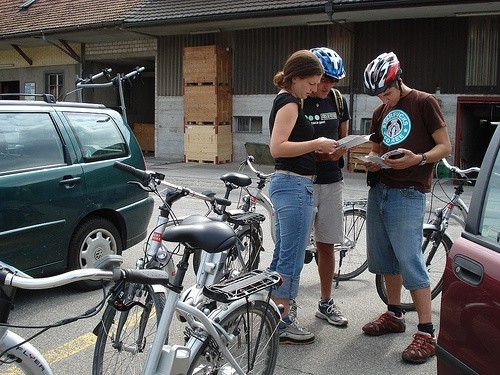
[275,169,317,182]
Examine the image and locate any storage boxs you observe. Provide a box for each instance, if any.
[183,45,233,165]
[349,139,373,173]
[133,123,155,153]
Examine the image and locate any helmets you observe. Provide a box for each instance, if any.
[309,47,347,80]
[363,52,402,96]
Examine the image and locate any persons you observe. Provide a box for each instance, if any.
[267,50,338,344]
[362,53,452,364]
[290,47,349,329]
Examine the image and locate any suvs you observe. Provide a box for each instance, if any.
[0,88,153,309]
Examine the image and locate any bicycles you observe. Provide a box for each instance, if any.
[0,223,287,375]
[373,156,497,310]
[226,153,366,283]
[85,159,269,374]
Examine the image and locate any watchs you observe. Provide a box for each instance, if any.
[419,153,427,167]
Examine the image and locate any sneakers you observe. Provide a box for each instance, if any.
[401,331,438,363]
[362,311,406,336]
[278,315,315,344]
[315,299,348,326]
[288,299,302,324]
